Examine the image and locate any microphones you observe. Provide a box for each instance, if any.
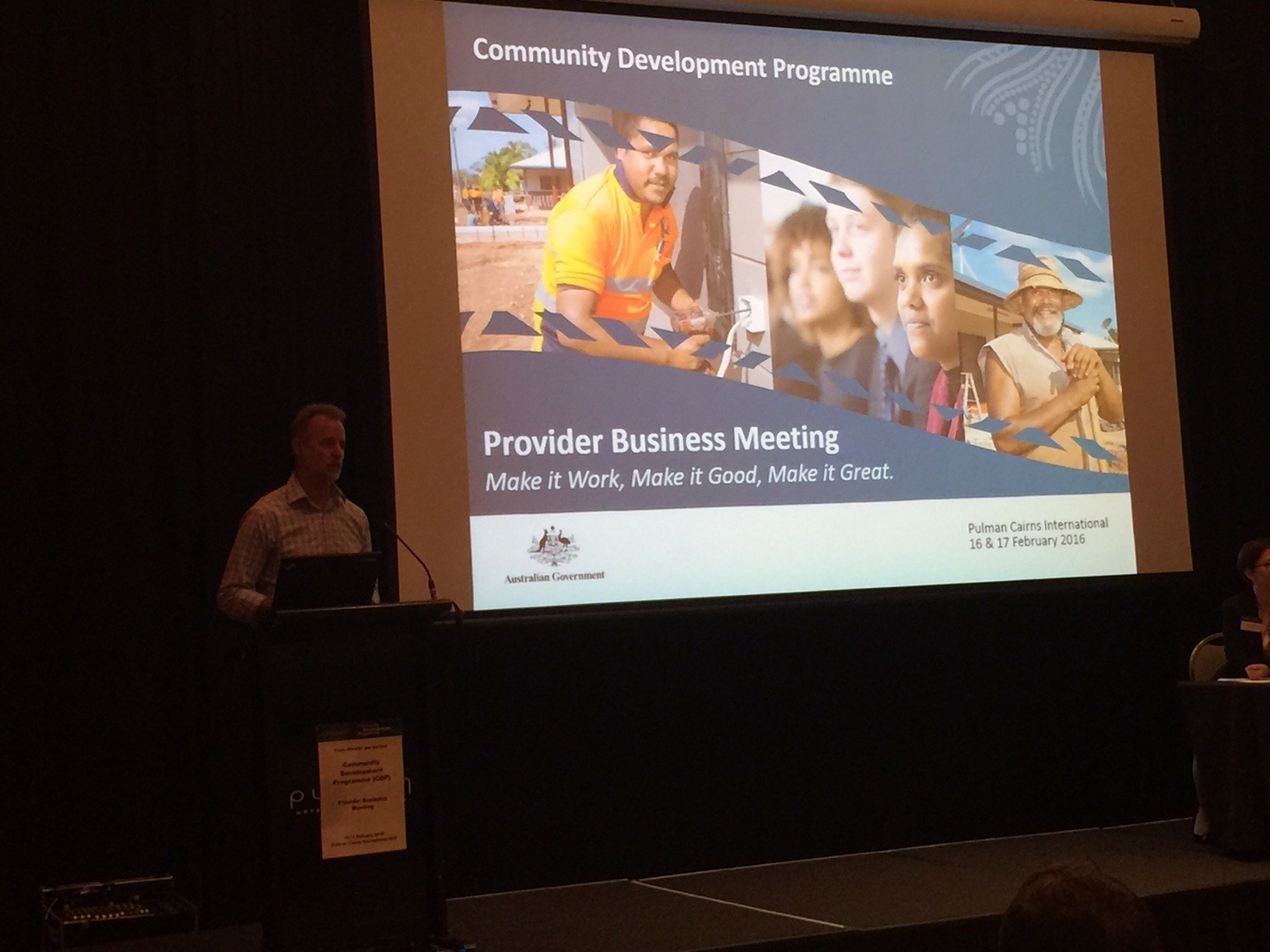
[372,512,438,602]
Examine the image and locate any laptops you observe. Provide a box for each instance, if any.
[272,552,381,609]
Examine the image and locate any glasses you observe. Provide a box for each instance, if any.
[1253,562,1270,569]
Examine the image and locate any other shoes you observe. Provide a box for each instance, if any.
[1194,833,1208,845]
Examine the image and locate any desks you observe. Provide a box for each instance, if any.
[1178,680,1270,861]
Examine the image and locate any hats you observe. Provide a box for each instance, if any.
[1002,255,1084,315]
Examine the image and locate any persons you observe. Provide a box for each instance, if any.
[766,173,964,444]
[530,113,713,371]
[209,403,380,628]
[461,185,505,222]
[997,862,1164,952]
[976,255,1124,473]
[1222,538,1270,679]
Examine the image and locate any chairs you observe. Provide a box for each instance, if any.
[1187,631,1228,682]
